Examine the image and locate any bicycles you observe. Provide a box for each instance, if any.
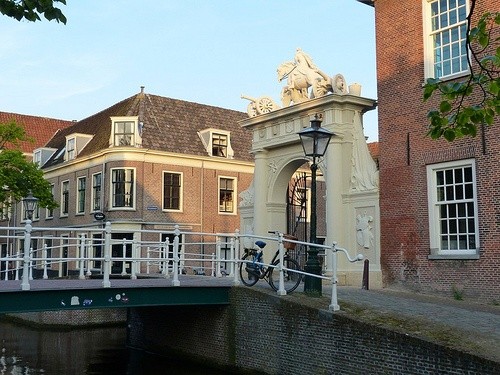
[240,231,302,294]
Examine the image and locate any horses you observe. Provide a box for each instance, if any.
[277,60,312,99]
[295,48,330,99]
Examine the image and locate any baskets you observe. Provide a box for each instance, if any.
[283,233,298,250]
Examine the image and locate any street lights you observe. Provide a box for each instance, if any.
[296,113,336,297]
[23,190,39,222]
